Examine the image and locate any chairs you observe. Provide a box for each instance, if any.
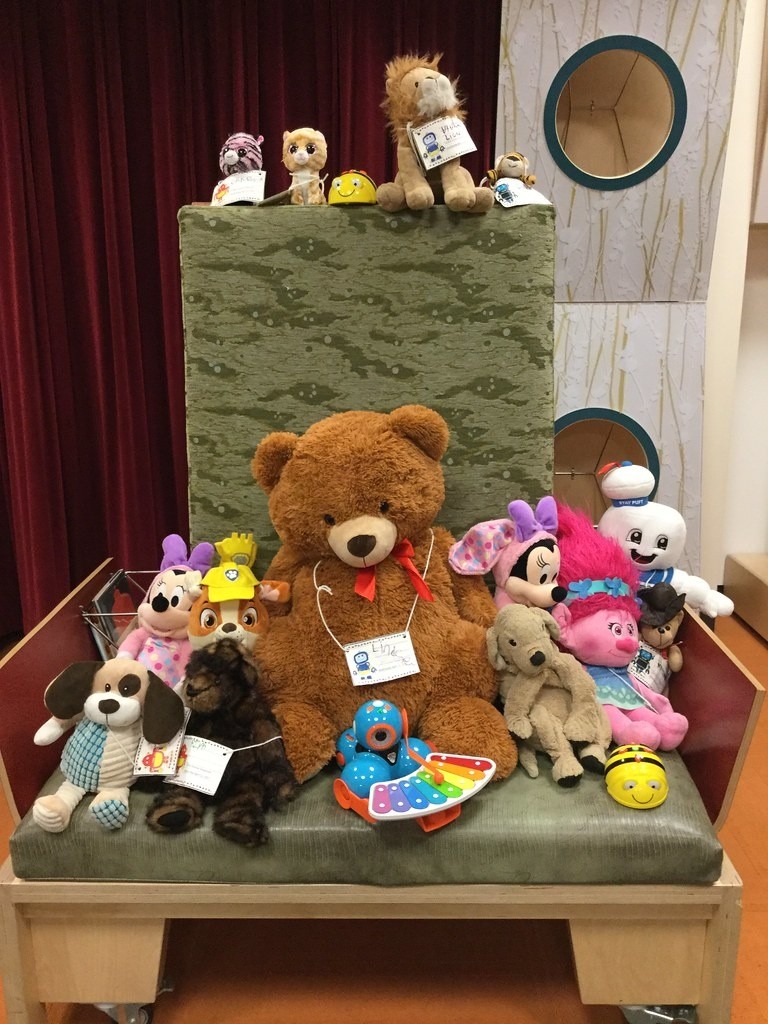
[0,199,767,1024]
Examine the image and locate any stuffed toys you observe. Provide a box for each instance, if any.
[219,131,263,176]
[117,404,734,848]
[375,52,495,214]
[486,151,536,188]
[32,659,184,834]
[283,127,327,206]
